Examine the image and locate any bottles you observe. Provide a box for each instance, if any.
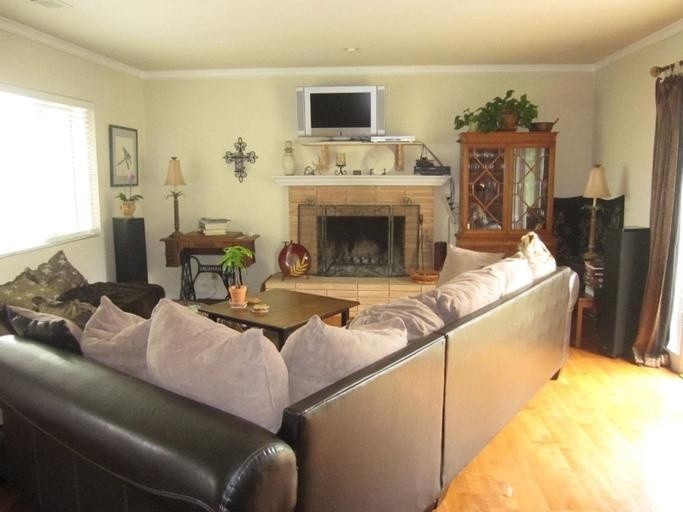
[283,153,296,174]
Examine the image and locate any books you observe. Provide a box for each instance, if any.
[198,217,230,236]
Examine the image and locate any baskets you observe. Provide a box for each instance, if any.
[408,267,440,284]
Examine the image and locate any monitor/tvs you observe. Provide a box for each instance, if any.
[296,85,385,141]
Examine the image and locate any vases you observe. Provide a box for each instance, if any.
[281,140,295,174]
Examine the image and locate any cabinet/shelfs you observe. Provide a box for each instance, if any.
[112,218,148,284]
[456,131,558,260]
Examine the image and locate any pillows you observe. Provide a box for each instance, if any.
[3,303,81,357]
[33,296,96,329]
[80,293,152,385]
[144,297,290,437]
[279,313,409,405]
[343,232,557,347]
[0,272,59,334]
[27,249,89,297]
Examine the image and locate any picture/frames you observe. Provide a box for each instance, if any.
[108,124,138,187]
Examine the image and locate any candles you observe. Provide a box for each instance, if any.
[335,152,346,166]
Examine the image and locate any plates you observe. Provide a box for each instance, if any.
[277,244,309,277]
[228,299,247,307]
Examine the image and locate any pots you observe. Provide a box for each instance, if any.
[527,118,559,131]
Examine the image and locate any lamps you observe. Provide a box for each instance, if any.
[581,164,611,251]
[163,155,187,238]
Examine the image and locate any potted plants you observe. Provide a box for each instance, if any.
[452,88,538,131]
[216,245,254,308]
[114,192,143,218]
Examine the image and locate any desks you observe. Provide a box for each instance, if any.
[159,231,259,302]
[572,290,608,351]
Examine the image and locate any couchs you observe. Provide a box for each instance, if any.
[0,265,581,511]
